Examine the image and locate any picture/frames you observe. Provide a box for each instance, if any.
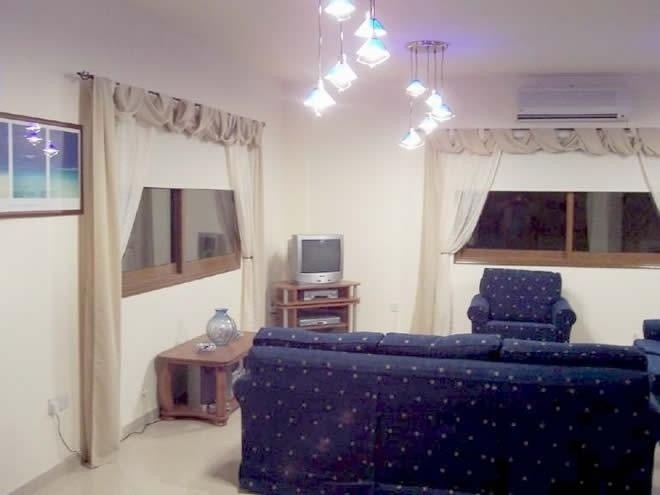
[1,111,85,221]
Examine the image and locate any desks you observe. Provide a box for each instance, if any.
[155,330,257,426]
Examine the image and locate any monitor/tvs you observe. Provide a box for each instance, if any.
[288,235,344,283]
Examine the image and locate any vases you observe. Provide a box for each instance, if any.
[206,307,238,347]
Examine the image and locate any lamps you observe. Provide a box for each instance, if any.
[399,39,457,154]
[301,0,390,116]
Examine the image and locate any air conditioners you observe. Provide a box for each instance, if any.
[515,86,631,123]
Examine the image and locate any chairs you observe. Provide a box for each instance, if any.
[632,319,660,406]
[466,267,577,344]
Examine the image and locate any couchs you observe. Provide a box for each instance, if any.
[231,324,660,495]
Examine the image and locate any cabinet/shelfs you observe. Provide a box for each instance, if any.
[273,280,362,334]
